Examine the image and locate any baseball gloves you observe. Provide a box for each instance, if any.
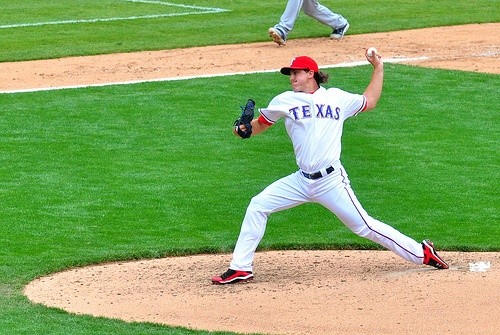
[232,99,256,139]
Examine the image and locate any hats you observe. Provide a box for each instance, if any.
[281,56,318,76]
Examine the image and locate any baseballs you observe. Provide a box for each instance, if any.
[367,47,378,57]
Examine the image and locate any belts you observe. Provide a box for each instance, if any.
[299,166,334,180]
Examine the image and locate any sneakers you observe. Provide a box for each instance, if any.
[330,21,349,40]
[421,239,449,269]
[268,24,288,46]
[211,268,254,285]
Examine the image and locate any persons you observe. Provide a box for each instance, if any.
[268,0,349,46]
[212,48,448,286]
